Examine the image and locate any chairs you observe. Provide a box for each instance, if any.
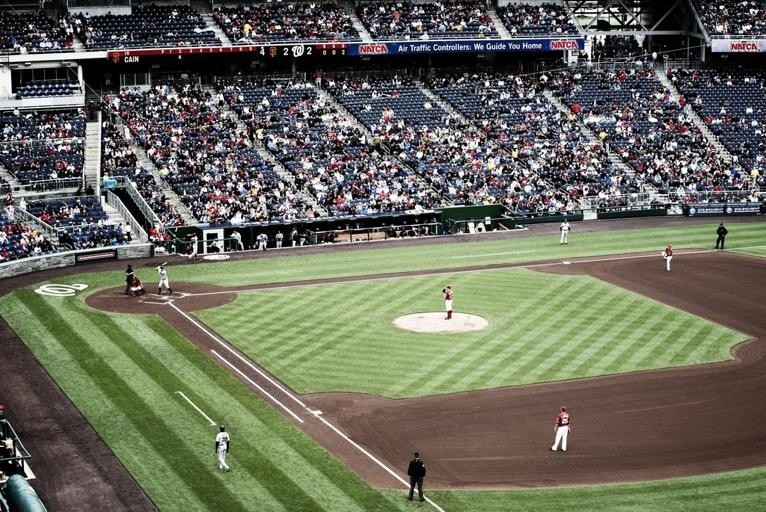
[0,0,766,264]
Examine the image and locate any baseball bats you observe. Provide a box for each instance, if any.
[155,261,168,271]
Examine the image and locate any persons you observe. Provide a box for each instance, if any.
[664,244,673,272]
[131,276,145,296]
[0,0,766,270]
[406,451,427,502]
[213,424,230,471]
[154,263,173,296]
[441,286,453,320]
[124,264,135,295]
[550,406,571,452]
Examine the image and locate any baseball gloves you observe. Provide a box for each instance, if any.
[443,289,446,292]
